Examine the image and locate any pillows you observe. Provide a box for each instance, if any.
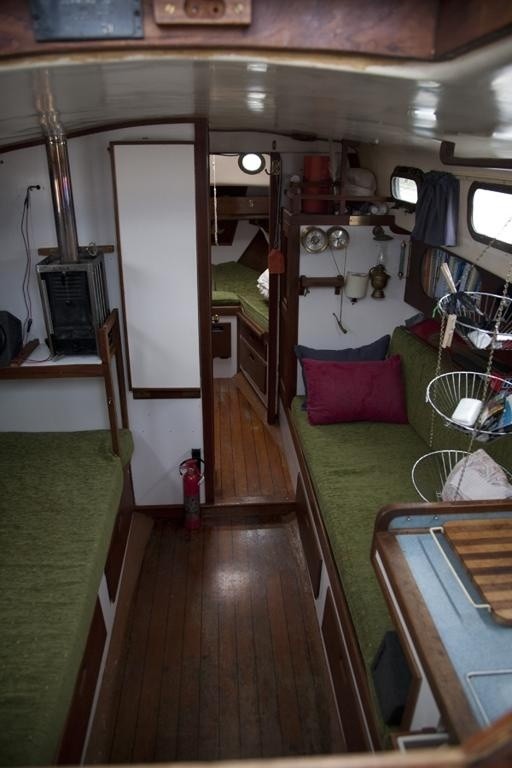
[293,334,409,425]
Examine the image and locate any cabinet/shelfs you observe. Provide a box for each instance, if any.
[237,311,268,409]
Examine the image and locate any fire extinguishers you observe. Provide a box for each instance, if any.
[178,457,208,530]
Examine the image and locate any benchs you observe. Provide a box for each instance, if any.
[291,323,512,751]
[0,429,136,768]
[211,260,270,333]
